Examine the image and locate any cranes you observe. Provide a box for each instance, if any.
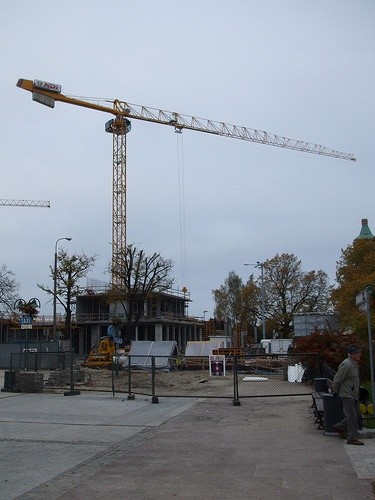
[16,78,356,294]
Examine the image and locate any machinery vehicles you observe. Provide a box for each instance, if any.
[81,336,117,368]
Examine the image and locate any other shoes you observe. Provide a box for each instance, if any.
[336,424,347,439]
[347,440,364,445]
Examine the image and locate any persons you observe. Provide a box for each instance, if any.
[288,345,292,351]
[332,345,364,445]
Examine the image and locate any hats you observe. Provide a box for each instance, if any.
[349,346,361,352]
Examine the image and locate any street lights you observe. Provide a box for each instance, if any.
[52,237,72,341]
[14,298,41,371]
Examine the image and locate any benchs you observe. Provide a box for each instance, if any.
[311,391,328,430]
